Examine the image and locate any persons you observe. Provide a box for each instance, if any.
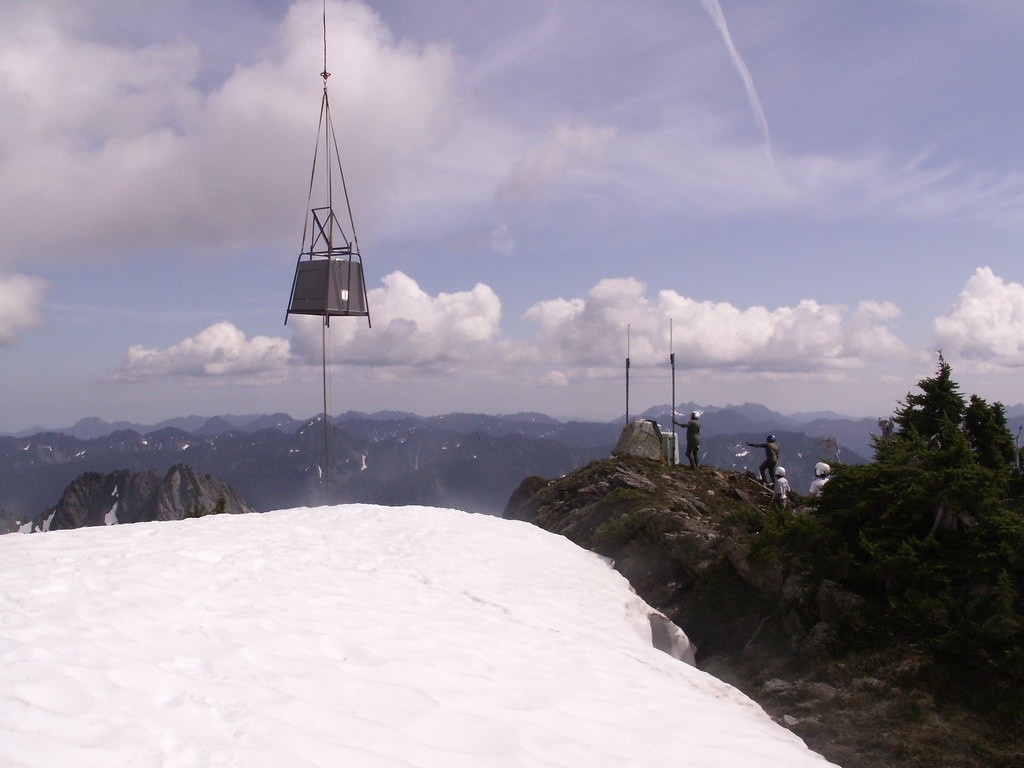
[673,411,701,471]
[809,462,830,499]
[746,434,780,488]
[771,466,791,512]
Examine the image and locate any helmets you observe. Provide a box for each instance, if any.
[692,412,700,418]
[767,434,775,442]
[815,462,830,475]
[774,467,785,476]
